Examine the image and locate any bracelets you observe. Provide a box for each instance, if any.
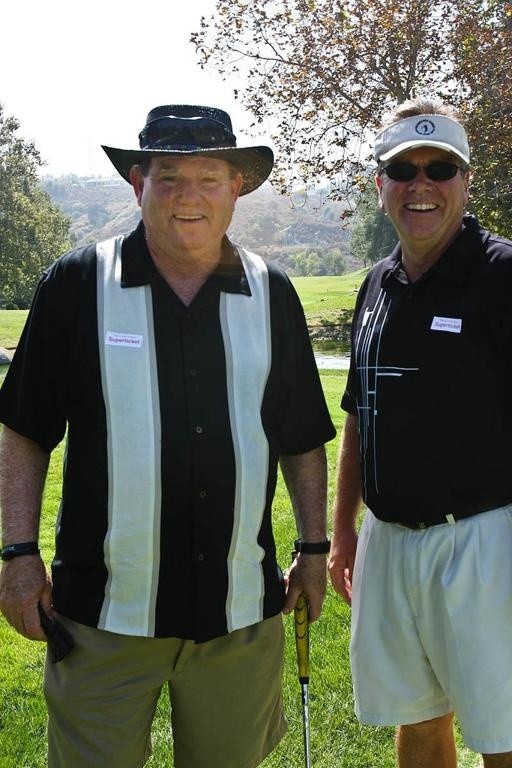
[0,541,42,562]
[291,534,331,557]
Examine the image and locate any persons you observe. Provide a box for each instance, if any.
[1,101,332,766]
[319,93,512,767]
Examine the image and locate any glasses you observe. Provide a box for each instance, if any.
[378,161,466,182]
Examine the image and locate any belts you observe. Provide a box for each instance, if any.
[398,499,512,531]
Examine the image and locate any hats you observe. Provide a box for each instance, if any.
[100,105,275,197]
[372,113,471,165]
